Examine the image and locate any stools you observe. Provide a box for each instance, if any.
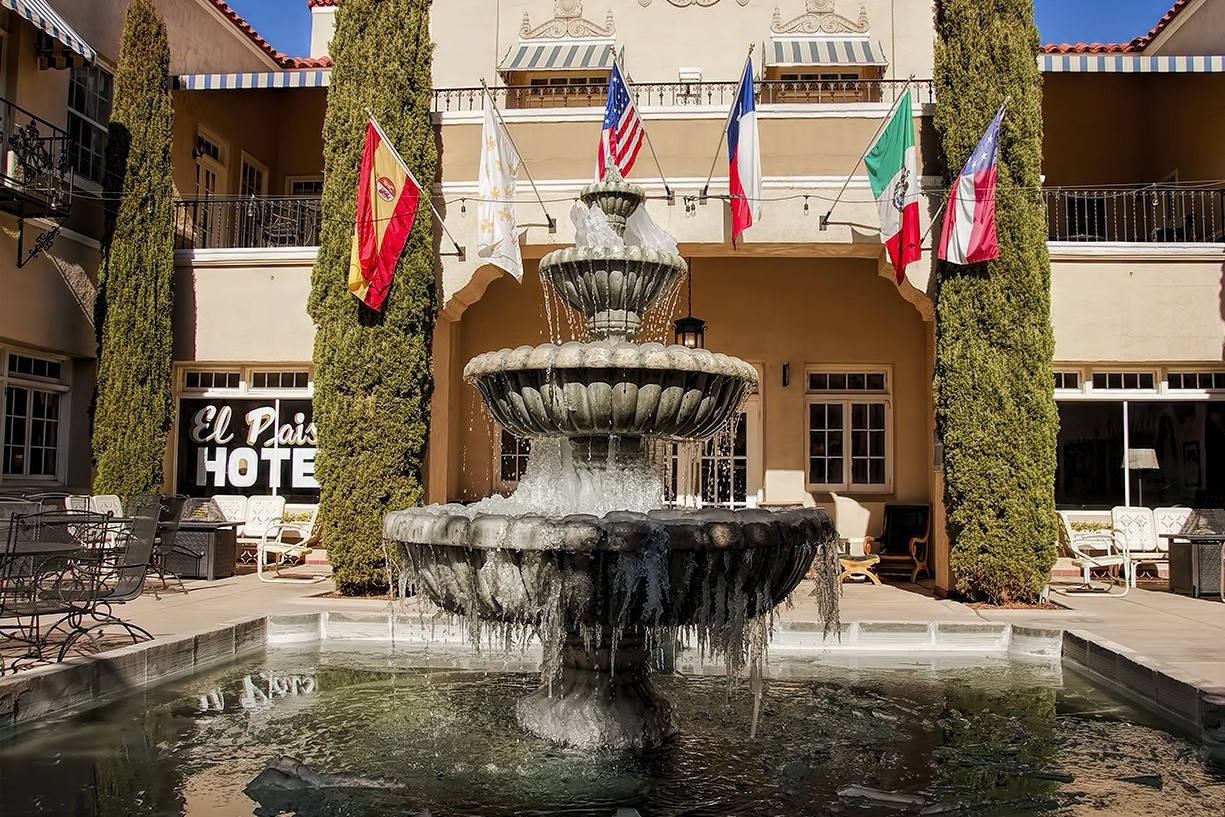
[837,553,883,586]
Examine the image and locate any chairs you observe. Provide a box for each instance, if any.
[755,469,816,509]
[251,195,321,247]
[1056,512,1130,598]
[0,493,206,679]
[1110,506,1194,582]
[1152,213,1197,242]
[865,502,932,583]
[257,503,327,583]
[208,495,287,567]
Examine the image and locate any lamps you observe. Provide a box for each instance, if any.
[1121,449,1160,507]
[671,258,708,349]
[192,136,206,160]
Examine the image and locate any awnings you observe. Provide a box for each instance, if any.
[0,0,96,70]
[496,45,624,88]
[762,40,890,84]
[1036,55,1225,72]
[165,72,333,91]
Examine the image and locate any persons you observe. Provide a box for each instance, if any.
[603,156,628,184]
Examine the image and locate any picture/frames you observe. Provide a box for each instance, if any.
[1183,441,1202,488]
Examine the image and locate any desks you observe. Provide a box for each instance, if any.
[1159,534,1225,598]
[157,521,246,581]
[0,517,136,527]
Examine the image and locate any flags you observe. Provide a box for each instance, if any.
[863,86,921,287]
[725,57,762,252]
[477,88,524,285]
[594,57,646,183]
[937,105,1007,265]
[348,115,421,314]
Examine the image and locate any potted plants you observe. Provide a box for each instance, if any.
[281,511,324,546]
[1066,520,1115,558]
